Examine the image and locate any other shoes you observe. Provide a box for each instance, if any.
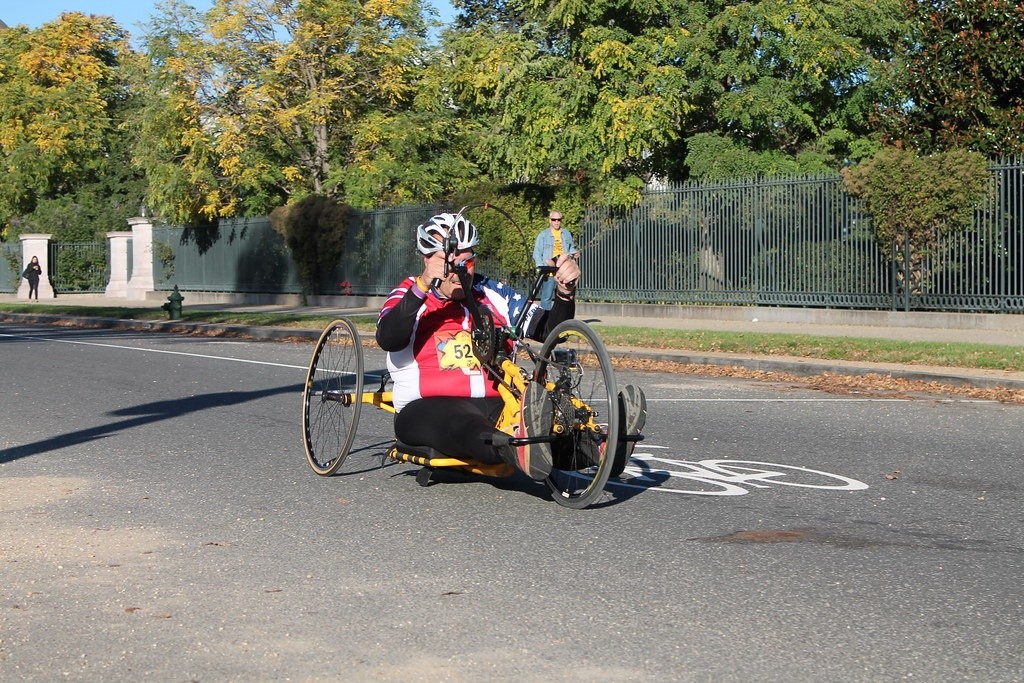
[28,298,33,303]
[34,298,39,302]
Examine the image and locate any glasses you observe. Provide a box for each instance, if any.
[551,218,562,222]
[451,253,476,270]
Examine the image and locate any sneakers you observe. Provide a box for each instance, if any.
[516,381,555,479]
[599,384,648,475]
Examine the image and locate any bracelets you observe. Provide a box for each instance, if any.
[411,275,429,299]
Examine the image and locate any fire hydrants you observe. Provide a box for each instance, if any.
[161,284,185,320]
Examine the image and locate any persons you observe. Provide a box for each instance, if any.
[531,210,578,310]
[26,256,42,302]
[374,212,647,481]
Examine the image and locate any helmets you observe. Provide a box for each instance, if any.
[416,212,480,256]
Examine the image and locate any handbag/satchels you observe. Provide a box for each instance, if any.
[22,268,30,279]
